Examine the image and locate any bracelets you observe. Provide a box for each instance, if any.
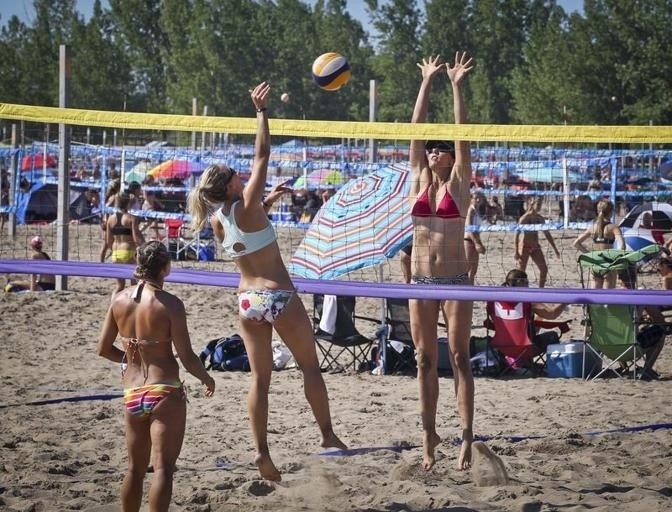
[255,106,267,112]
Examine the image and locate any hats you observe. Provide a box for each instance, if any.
[424,140,455,153]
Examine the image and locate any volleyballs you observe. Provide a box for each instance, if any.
[311,52,352,91]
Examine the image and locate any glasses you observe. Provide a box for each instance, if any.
[227,168,237,182]
[426,143,450,154]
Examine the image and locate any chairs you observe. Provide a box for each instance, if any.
[166,220,200,261]
[308,244,664,380]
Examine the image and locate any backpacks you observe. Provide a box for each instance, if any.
[198,334,250,372]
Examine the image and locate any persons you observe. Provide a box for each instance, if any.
[291,189,336,221]
[465,192,485,280]
[471,174,671,222]
[573,199,671,378]
[1,157,213,293]
[96,240,215,512]
[400,239,414,282]
[405,50,476,472]
[487,200,568,364]
[186,81,348,483]
